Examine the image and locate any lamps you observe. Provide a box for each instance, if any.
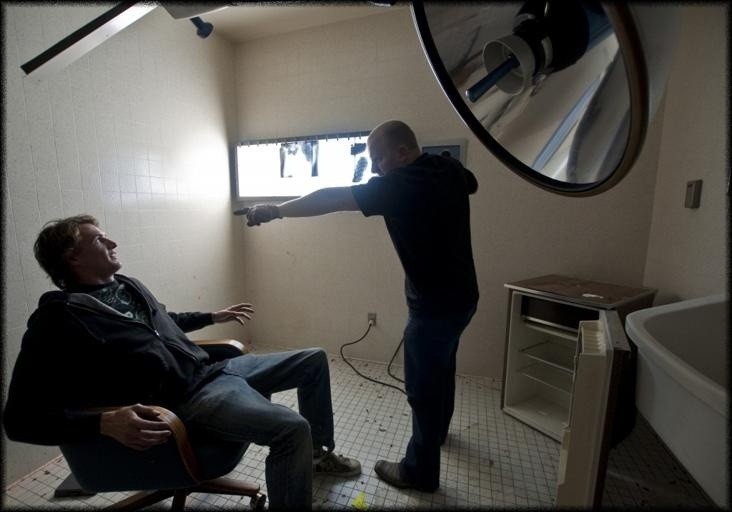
[409,1,679,200]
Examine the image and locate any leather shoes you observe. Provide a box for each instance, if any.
[374,460,438,493]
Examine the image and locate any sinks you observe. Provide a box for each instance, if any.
[625,293,732,508]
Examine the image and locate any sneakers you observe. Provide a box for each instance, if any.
[311,451,360,476]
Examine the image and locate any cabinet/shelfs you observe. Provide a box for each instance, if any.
[502,273,659,510]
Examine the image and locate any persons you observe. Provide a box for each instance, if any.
[1,212,362,511]
[234,119,480,494]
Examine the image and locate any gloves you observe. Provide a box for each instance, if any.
[233,205,281,227]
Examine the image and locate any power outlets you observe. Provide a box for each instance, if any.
[368,312,376,325]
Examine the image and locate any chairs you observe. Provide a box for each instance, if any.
[58,335,265,512]
